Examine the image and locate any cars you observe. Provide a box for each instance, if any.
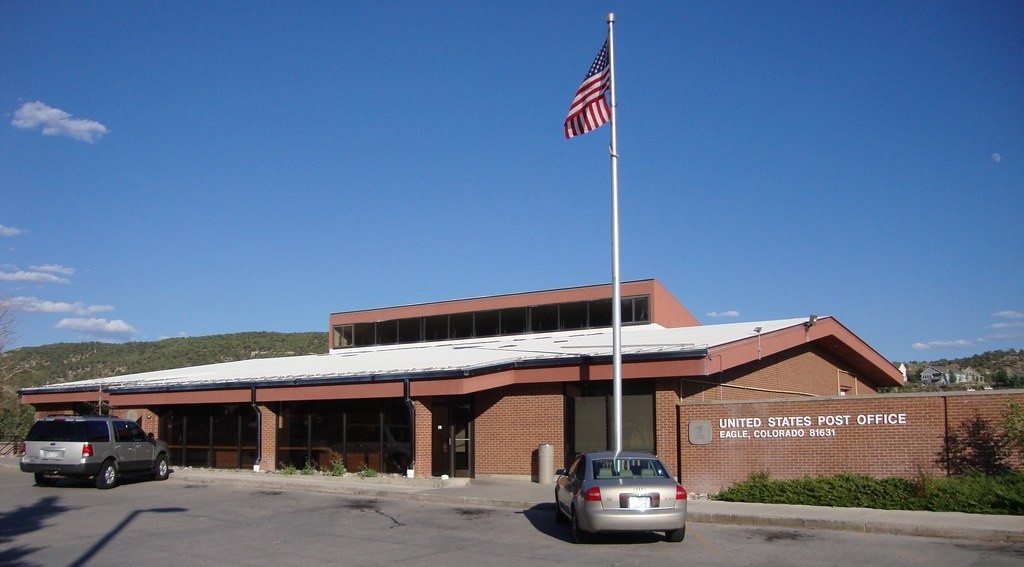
[554,451,688,543]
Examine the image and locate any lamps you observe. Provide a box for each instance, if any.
[803,314,817,326]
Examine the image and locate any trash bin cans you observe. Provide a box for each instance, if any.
[538,444,555,484]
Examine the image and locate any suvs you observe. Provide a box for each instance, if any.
[16,414,172,491]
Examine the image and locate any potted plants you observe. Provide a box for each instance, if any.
[406,461,414,478]
[253,456,260,471]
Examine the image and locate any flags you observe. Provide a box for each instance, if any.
[564,37,611,139]
